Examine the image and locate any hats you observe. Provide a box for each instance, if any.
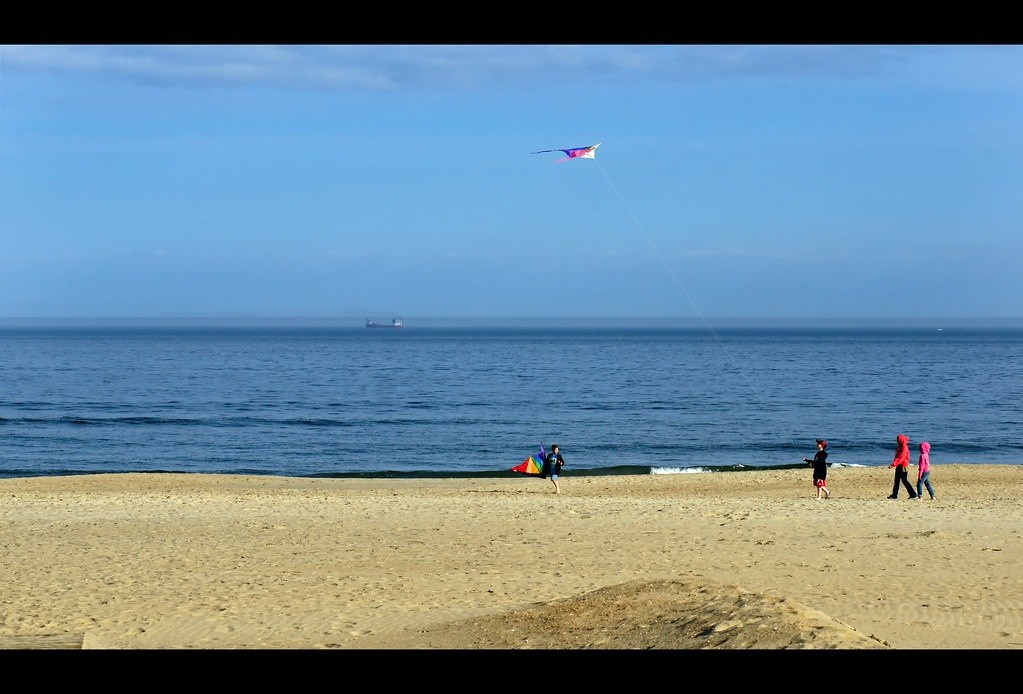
[816,438,827,450]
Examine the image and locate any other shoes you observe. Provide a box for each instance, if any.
[908,494,918,500]
[887,496,897,499]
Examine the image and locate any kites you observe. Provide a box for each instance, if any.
[531,143,602,163]
[510,441,547,474]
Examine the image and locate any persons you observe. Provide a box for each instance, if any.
[545,445,565,494]
[886,435,918,499]
[916,442,936,500]
[802,439,831,501]
